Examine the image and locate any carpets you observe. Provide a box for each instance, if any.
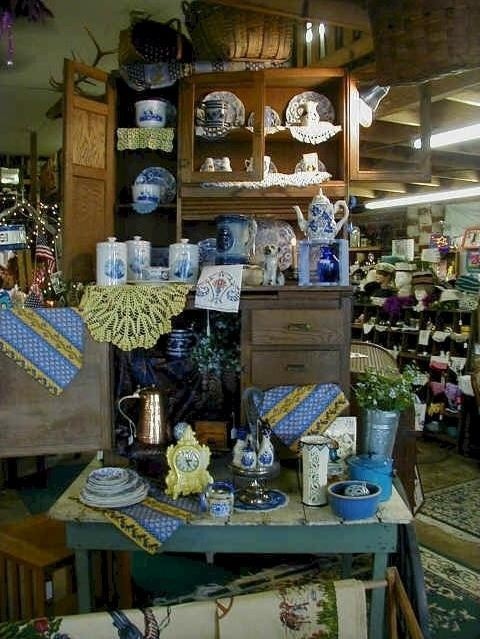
[150,540,480,639]
[412,477,480,544]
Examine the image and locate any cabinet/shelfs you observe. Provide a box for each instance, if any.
[0,58,480,487]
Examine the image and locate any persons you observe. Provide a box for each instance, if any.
[373,261,399,293]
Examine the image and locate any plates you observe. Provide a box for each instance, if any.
[78,467,148,510]
[151,247,169,267]
[254,220,296,271]
[135,168,176,202]
[195,239,216,271]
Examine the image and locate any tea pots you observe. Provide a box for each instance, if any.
[292,187,350,239]
[296,101,321,124]
[116,387,176,443]
[199,482,234,518]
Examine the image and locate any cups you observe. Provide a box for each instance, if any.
[165,328,194,358]
[244,156,271,174]
[202,157,232,172]
[134,99,168,129]
[195,100,227,127]
[214,213,257,264]
[300,436,332,505]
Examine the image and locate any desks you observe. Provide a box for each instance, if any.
[44,454,413,637]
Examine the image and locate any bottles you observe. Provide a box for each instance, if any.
[124,236,152,281]
[318,246,338,282]
[264,105,271,127]
[168,238,199,282]
[233,426,275,470]
[95,235,127,286]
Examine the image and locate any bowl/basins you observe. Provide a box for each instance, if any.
[130,184,160,202]
[328,480,382,520]
[145,267,171,281]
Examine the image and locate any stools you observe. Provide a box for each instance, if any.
[2,510,103,621]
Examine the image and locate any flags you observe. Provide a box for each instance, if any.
[37,225,55,274]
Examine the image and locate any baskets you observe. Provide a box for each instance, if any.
[181,0,294,64]
[118,16,197,66]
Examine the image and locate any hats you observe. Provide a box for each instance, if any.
[376,262,395,274]
[412,272,433,284]
